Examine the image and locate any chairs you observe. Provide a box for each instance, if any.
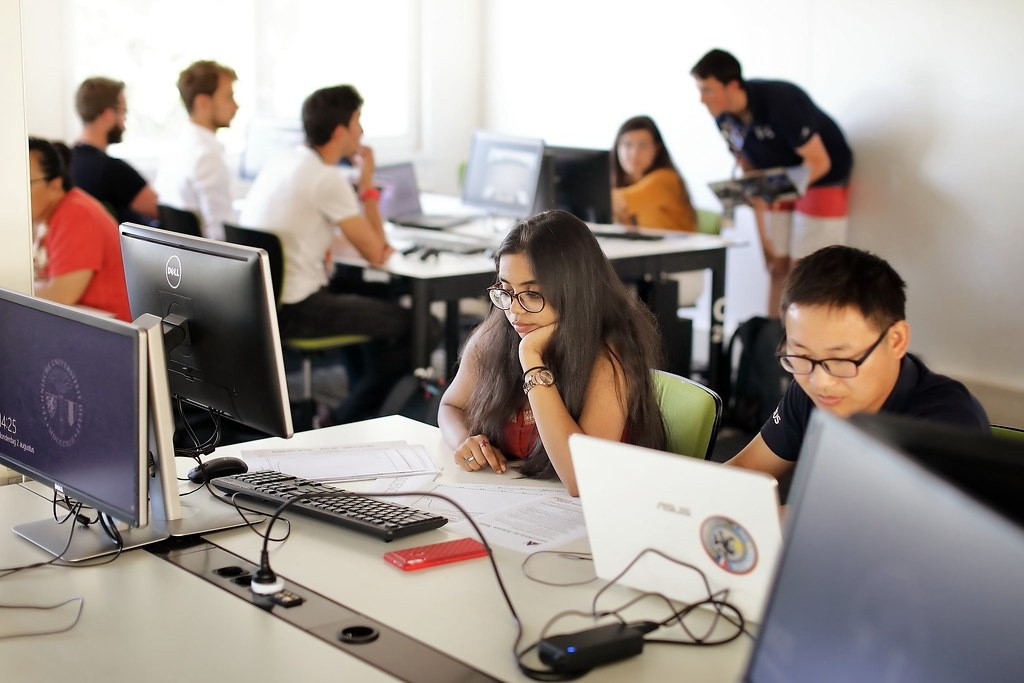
[224,223,378,432]
[678,208,721,308]
[155,204,203,238]
[649,368,723,460]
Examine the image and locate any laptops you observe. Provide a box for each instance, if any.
[709,164,813,210]
[569,432,782,626]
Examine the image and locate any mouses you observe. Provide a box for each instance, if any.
[187,457,248,485]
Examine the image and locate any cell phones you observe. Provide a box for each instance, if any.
[383,537,492,572]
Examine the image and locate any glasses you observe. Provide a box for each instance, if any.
[486,281,545,313]
[776,322,892,379]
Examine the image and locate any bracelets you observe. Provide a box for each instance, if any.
[359,188,381,203]
[523,366,550,383]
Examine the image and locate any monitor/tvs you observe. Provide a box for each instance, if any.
[118,220,294,537]
[461,133,613,225]
[0,287,171,563]
[738,405,1024,683]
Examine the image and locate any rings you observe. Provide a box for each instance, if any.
[481,443,490,447]
[466,456,474,463]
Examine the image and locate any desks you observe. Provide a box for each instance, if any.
[1,416,762,683]
[330,192,730,422]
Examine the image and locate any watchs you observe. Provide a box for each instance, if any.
[522,370,555,394]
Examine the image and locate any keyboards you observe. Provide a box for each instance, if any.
[406,236,490,254]
[210,469,449,543]
[593,231,663,241]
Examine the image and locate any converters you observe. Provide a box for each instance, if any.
[538,621,644,673]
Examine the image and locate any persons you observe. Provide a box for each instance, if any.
[437,209,676,497]
[160,61,239,243]
[239,86,441,429]
[720,246,993,509]
[602,118,697,232]
[69,77,157,225]
[690,49,854,330]
[30,137,137,327]
